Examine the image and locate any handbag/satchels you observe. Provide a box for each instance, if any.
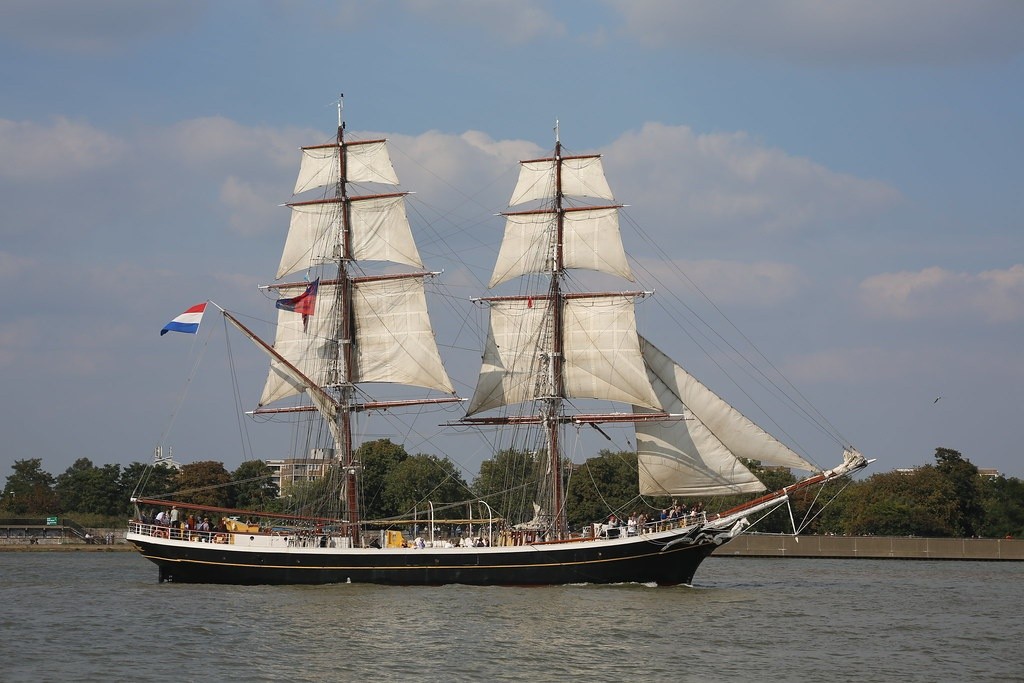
[197,525,203,531]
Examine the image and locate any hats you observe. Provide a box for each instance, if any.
[204,518,209,521]
[172,506,176,509]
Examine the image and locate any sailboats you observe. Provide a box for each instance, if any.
[125,92,880,586]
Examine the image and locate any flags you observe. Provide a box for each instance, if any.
[160,302,208,336]
[276,276,319,316]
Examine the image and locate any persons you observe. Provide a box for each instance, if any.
[661,502,702,531]
[30,535,35,544]
[609,516,617,528]
[402,540,407,548]
[444,535,492,548]
[141,505,215,542]
[298,530,315,546]
[106,532,114,544]
[412,538,425,549]
[369,534,379,548]
[409,523,513,539]
[628,512,653,536]
[85,533,94,544]
